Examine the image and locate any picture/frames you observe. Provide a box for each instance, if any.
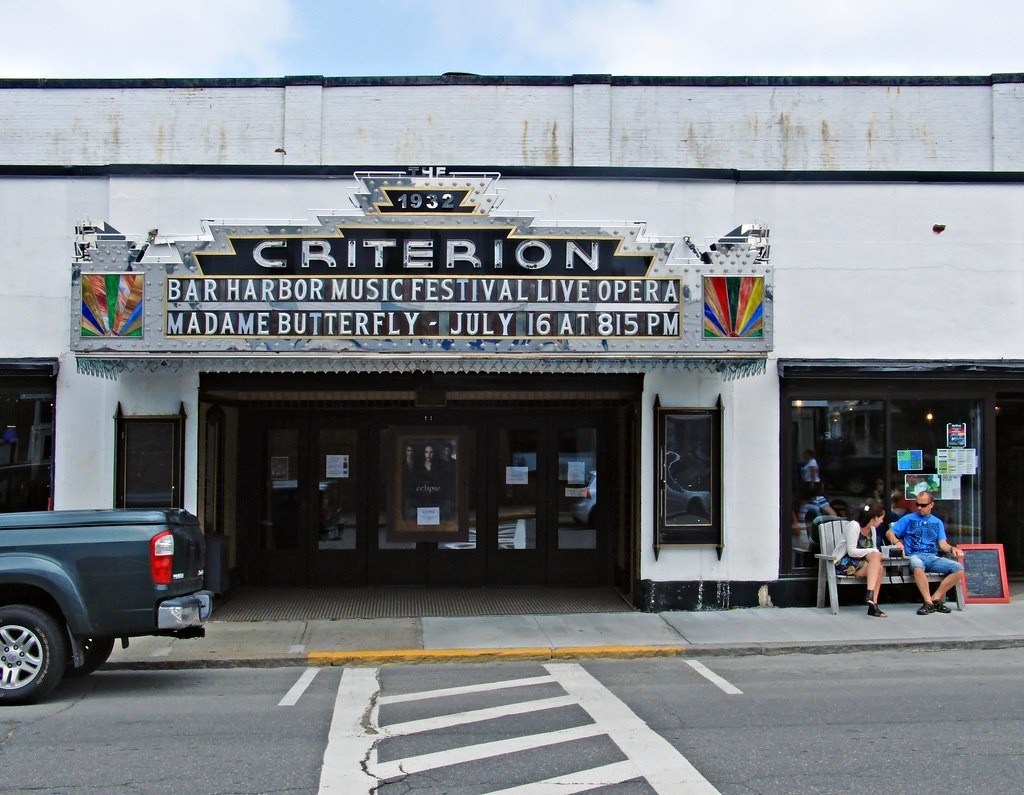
[384,424,469,542]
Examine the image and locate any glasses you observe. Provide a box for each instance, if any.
[916,501,932,507]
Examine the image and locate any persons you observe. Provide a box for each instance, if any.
[799,448,821,507]
[797,478,917,549]
[886,491,965,615]
[402,442,457,521]
[832,501,887,617]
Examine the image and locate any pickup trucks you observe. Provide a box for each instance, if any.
[274,478,344,550]
[0,508,213,706]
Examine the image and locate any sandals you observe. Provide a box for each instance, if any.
[933,600,951,613]
[917,602,935,615]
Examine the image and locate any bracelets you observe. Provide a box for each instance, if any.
[950,546,955,553]
[894,541,901,545]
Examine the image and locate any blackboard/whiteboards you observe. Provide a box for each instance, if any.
[956,543,1011,606]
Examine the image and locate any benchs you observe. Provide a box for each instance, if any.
[814,520,965,615]
[791,516,846,568]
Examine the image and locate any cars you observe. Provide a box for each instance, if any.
[570,471,598,530]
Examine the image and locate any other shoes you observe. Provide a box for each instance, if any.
[868,603,887,617]
[865,590,874,605]
